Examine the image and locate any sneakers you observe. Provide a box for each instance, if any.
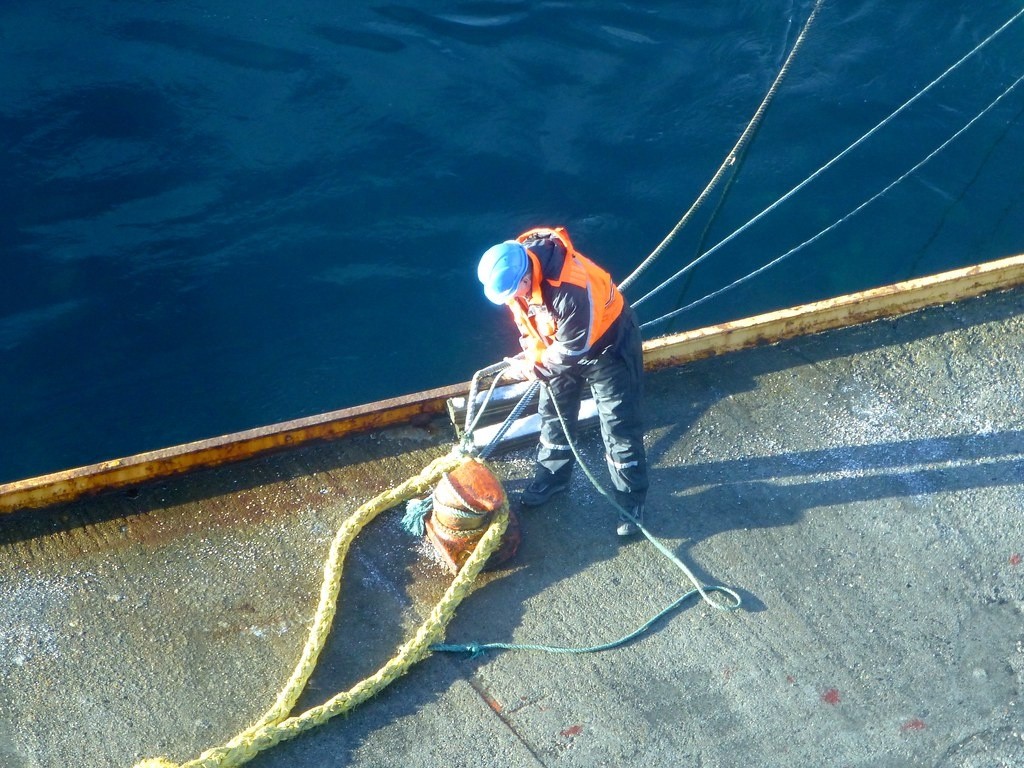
[616,503,644,536]
[521,480,571,505]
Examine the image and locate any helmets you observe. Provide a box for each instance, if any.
[478,239,528,306]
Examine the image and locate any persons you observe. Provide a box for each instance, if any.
[478,227,649,536]
